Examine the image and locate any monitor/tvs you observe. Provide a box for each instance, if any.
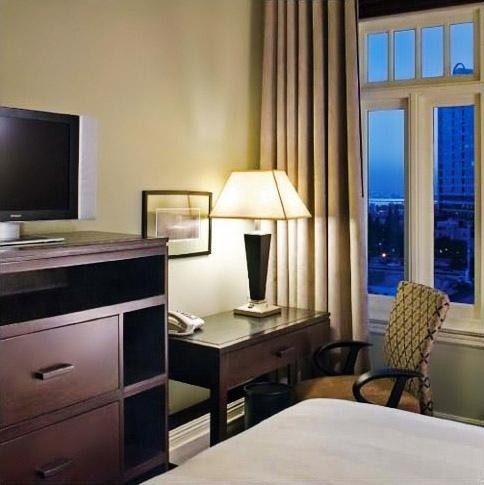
[0,105,80,246]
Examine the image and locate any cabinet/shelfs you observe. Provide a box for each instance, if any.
[0,232,169,484]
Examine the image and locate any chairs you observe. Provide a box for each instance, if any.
[293,280,450,416]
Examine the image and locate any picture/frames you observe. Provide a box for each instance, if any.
[142,191,212,259]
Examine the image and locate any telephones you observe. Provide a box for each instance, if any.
[167,310,205,333]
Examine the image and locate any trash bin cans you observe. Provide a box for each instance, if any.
[244,382,290,431]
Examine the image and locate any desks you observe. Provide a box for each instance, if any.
[165,302,331,445]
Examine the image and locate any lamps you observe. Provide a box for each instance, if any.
[207,169,312,319]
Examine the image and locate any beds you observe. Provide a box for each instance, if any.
[140,399,484,484]
